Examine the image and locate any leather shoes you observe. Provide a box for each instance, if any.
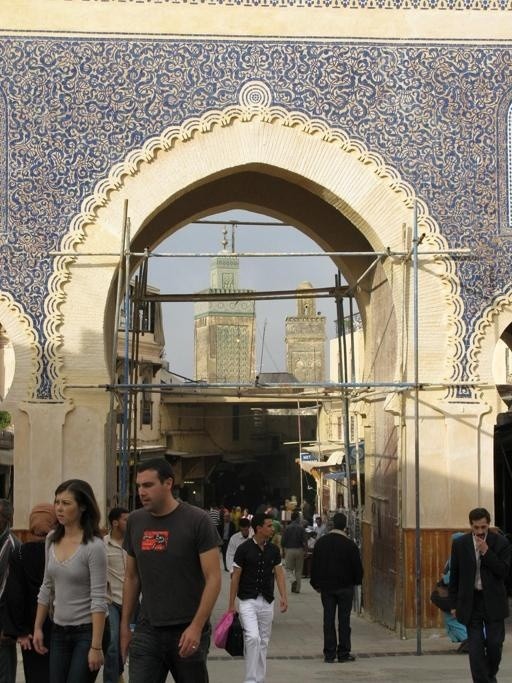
[324,657,335,663]
[338,655,355,662]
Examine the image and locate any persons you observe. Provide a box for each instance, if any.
[14,502,64,683]
[32,480,110,683]
[314,517,326,536]
[103,507,139,683]
[231,506,242,531]
[441,531,472,653]
[281,511,309,594]
[264,507,280,543]
[229,506,235,521]
[226,518,255,571]
[281,495,317,520]
[202,503,229,540]
[241,506,254,520]
[309,513,364,663]
[302,519,312,532]
[120,458,222,683]
[448,509,512,683]
[0,519,24,683]
[228,515,288,682]
[307,530,318,548]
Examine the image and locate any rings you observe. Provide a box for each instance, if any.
[192,645,197,650]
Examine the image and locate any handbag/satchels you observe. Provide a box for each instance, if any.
[225,612,243,657]
[215,611,234,648]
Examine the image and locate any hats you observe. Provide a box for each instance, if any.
[30,503,58,537]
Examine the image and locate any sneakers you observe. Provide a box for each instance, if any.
[456,640,469,654]
[119,673,125,683]
[291,581,297,592]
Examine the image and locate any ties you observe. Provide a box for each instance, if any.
[476,549,480,582]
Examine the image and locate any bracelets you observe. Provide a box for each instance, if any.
[89,645,103,651]
